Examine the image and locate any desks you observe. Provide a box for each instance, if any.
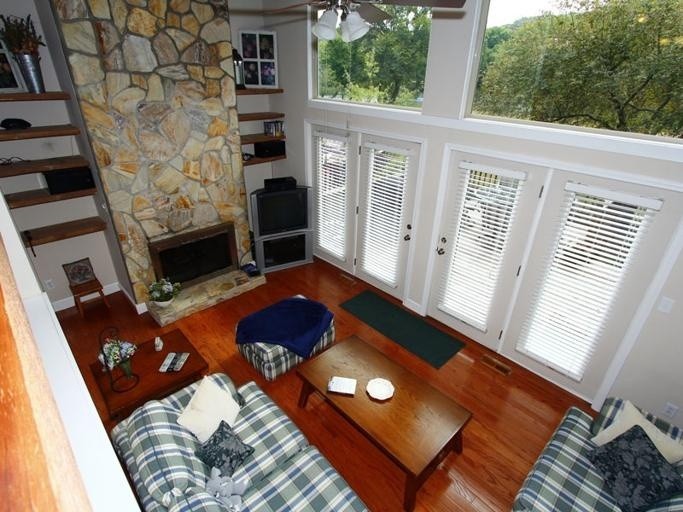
[295,334,474,512]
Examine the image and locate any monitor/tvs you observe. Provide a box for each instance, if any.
[254,231,314,274]
[250,184,312,243]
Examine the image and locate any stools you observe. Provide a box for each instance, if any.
[235,294,335,382]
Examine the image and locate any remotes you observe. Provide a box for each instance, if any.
[167,353,182,371]
[173,352,190,372]
[159,352,176,373]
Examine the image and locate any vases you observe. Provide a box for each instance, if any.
[116,358,132,381]
[154,295,175,308]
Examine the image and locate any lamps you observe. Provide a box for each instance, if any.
[310,1,371,44]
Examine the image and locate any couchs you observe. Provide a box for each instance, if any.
[111,372,377,512]
[511,396,682,512]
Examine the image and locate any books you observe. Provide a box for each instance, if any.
[326,375,356,396]
[158,351,189,373]
[263,121,275,137]
[279,121,284,136]
[274,121,279,137]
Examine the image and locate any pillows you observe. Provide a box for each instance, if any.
[193,419,256,478]
[177,373,243,445]
[589,400,681,466]
[586,424,682,512]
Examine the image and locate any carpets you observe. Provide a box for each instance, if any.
[339,289,467,369]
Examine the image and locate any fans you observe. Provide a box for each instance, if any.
[260,0,393,23]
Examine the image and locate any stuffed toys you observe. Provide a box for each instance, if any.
[203,465,247,512]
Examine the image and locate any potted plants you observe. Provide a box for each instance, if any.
[1,14,47,93]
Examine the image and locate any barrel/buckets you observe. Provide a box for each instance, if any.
[12,53,45,94]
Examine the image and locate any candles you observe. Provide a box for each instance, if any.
[232,59,245,89]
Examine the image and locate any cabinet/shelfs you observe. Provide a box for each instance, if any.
[236,87,287,168]
[0,92,107,249]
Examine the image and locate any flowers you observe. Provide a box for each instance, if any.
[144,278,182,302]
[94,325,137,373]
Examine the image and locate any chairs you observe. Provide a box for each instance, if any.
[62,257,111,320]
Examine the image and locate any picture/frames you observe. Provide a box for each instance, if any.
[0,41,23,91]
[238,30,278,89]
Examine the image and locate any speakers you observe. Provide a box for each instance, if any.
[254,140,285,158]
[45,167,95,196]
